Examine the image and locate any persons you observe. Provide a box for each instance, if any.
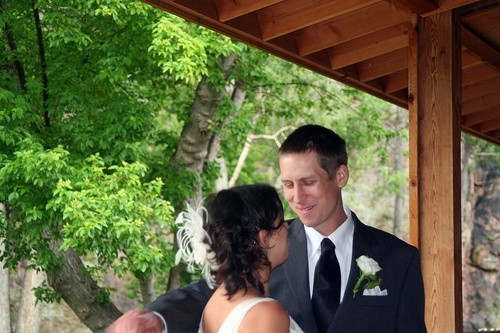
[177,184,305,333]
[106,124,428,333]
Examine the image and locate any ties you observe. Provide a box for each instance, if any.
[311,238,341,333]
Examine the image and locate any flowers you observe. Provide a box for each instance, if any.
[352,253,382,298]
